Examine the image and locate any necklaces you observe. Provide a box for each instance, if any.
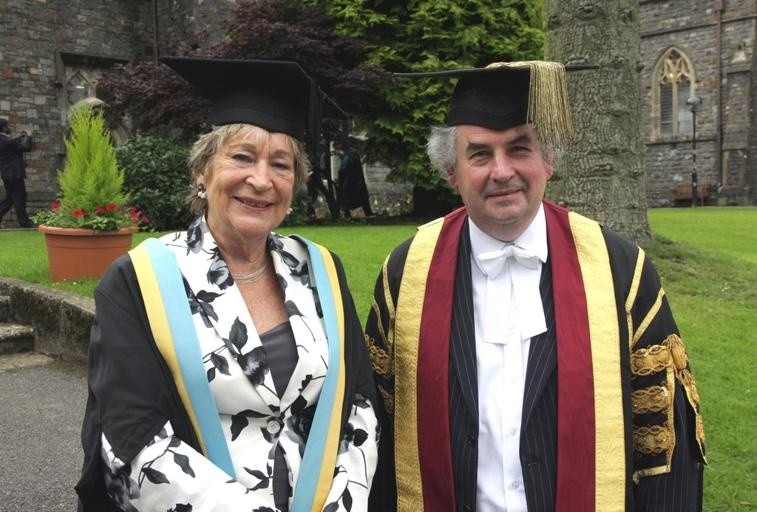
[231,261,270,284]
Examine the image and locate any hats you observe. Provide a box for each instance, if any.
[392,66,602,131]
[159,56,350,142]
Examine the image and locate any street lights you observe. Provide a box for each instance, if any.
[685,95,704,208]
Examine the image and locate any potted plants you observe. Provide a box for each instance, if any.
[35,99,137,287]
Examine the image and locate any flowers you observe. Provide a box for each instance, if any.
[52,198,150,228]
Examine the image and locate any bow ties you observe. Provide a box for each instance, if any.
[477,245,548,345]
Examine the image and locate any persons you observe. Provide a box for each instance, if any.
[364,63,707,512]
[1,115,36,229]
[76,81,382,512]
